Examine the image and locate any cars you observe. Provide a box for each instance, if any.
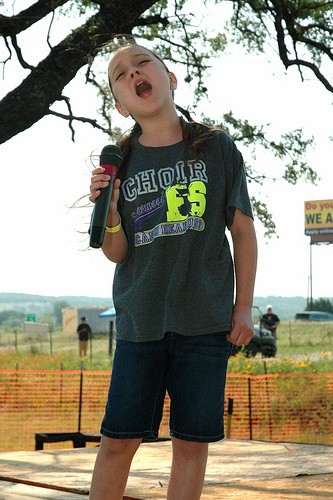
[246,326,277,358]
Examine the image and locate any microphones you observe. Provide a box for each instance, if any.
[90,144,122,248]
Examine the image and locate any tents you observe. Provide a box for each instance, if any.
[99,308,117,318]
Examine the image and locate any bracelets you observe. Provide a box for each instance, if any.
[105,211,121,233]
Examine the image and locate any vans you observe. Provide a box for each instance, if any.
[294,311,333,321]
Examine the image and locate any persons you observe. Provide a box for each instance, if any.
[90,45,259,500]
[77,317,93,357]
[259,305,280,340]
